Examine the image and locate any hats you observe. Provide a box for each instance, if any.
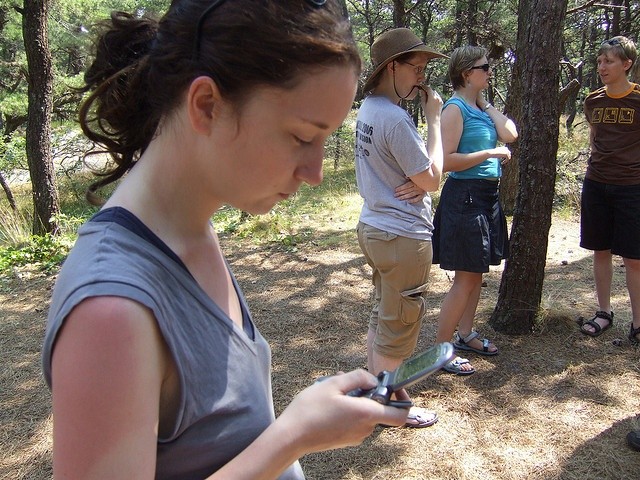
[363,27,450,90]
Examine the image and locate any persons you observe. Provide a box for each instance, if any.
[355,27,444,428]
[579,36,640,347]
[41,0,411,480]
[437,47,519,375]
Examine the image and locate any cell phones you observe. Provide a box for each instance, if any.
[315,341,454,407]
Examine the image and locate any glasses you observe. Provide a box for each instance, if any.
[184,1,337,57]
[601,39,630,59]
[401,61,427,74]
[471,64,491,71]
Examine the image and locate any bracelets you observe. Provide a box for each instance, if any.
[482,102,491,112]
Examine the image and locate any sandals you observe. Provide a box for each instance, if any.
[379,406,438,427]
[580,311,614,336]
[455,332,498,355]
[442,353,474,374]
